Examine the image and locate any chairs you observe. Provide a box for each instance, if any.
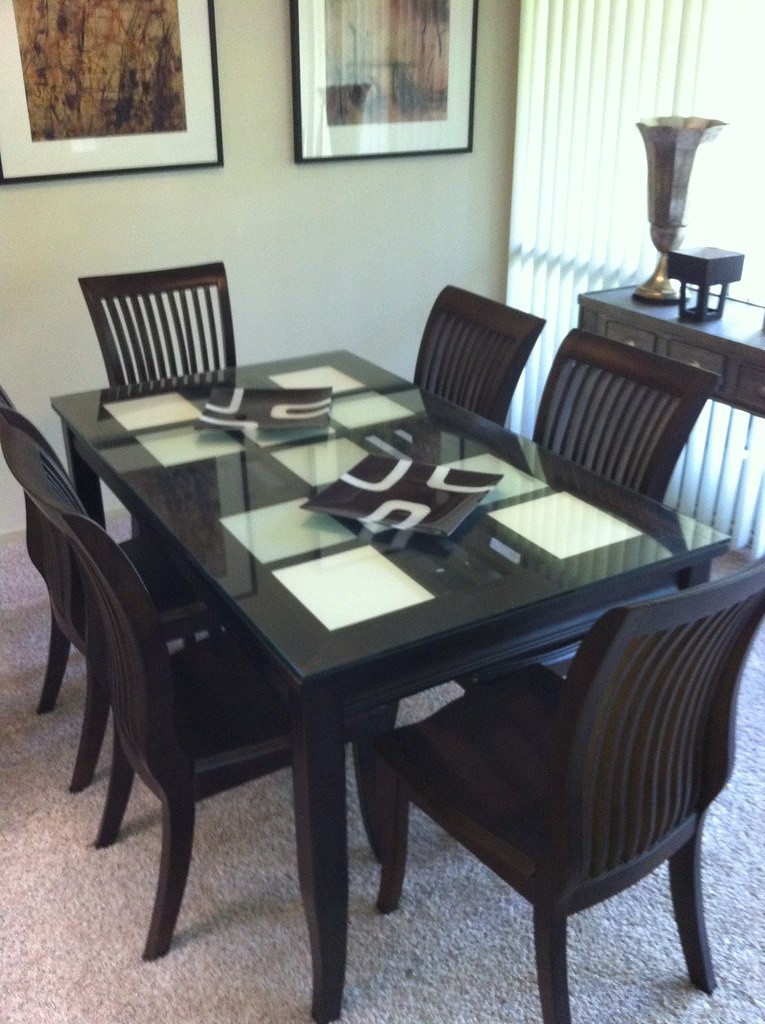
[412,285,546,427]
[376,549,764,1024]
[0,384,218,798]
[464,328,724,686]
[78,260,236,389]
[1,406,400,964]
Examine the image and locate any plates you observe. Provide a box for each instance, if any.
[194,386,333,429]
[300,453,504,538]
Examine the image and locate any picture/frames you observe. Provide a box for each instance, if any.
[0,0,226,185]
[289,0,478,163]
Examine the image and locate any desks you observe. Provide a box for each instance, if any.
[49,350,732,1024]
[578,284,765,421]
